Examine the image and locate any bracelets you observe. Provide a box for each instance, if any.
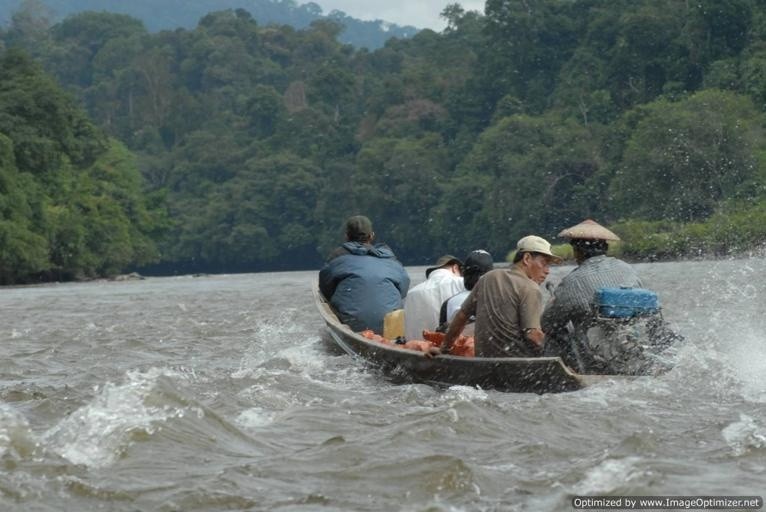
[439,342,455,354]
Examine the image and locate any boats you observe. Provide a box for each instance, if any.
[311,275,688,395]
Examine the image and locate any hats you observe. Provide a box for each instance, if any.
[345,212,375,242]
[515,234,564,264]
[461,248,495,270]
[424,254,466,278]
[555,216,622,243]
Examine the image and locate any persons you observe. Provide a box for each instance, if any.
[439,249,495,338]
[420,234,564,361]
[318,213,412,338]
[403,255,467,345]
[540,219,676,376]
